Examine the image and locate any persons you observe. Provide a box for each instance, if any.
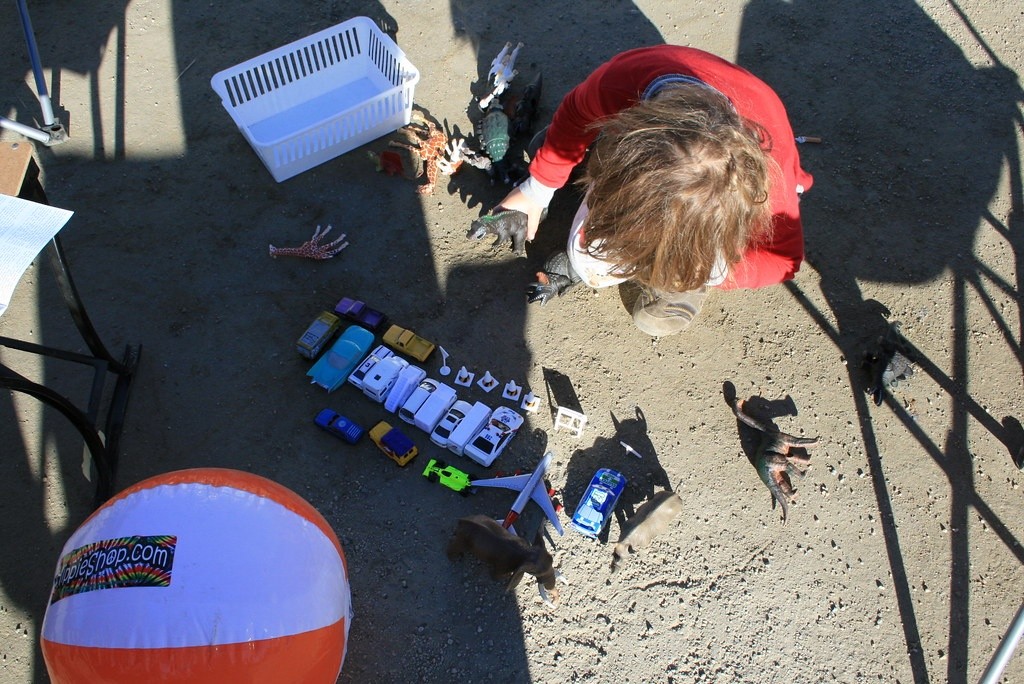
[491,44,813,337]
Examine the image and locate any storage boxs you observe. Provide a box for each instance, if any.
[210,15,421,182]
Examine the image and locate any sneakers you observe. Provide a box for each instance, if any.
[633,283,716,337]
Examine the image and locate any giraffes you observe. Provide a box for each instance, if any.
[268,225,350,260]
[388,113,448,196]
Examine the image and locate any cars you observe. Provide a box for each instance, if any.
[570,468,628,542]
[348,344,395,391]
[332,297,389,335]
[398,378,441,426]
[462,406,525,469]
[382,324,435,364]
[368,420,420,468]
[315,408,365,446]
[429,400,473,449]
[295,310,343,361]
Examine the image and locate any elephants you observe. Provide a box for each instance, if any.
[445,514,569,608]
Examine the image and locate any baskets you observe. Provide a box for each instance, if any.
[210,16,420,184]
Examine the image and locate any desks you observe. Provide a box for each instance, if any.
[0,140,144,516]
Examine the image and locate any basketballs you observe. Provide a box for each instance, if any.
[34,464,358,684]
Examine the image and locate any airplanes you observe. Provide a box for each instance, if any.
[470,450,565,537]
